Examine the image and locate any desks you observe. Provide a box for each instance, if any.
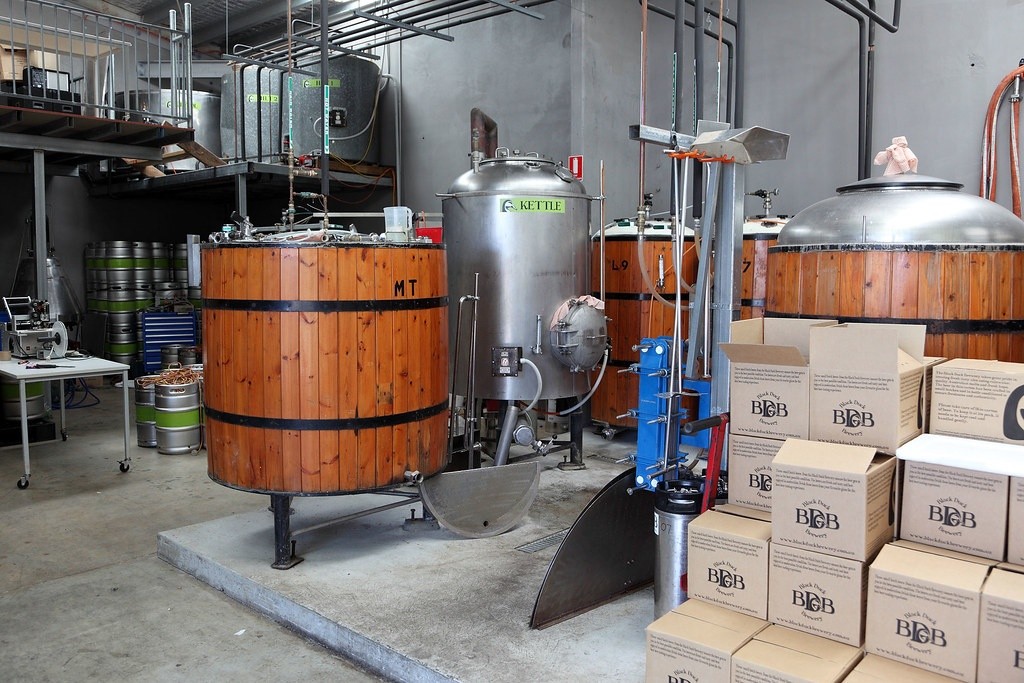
[0,353,130,490]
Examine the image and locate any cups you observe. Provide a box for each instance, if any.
[383,206,413,244]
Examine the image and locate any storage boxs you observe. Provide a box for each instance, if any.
[0,44,39,80]
[53,90,81,114]
[645,317,1024,683]
[7,86,53,111]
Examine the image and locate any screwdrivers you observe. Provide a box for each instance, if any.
[38,365,75,368]
